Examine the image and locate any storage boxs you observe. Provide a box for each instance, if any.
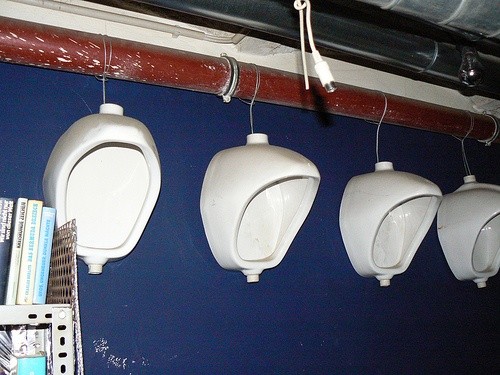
[9,353,46,375]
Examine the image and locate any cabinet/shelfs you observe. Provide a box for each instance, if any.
[0,303,76,375]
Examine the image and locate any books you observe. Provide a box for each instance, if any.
[0,198,56,375]
